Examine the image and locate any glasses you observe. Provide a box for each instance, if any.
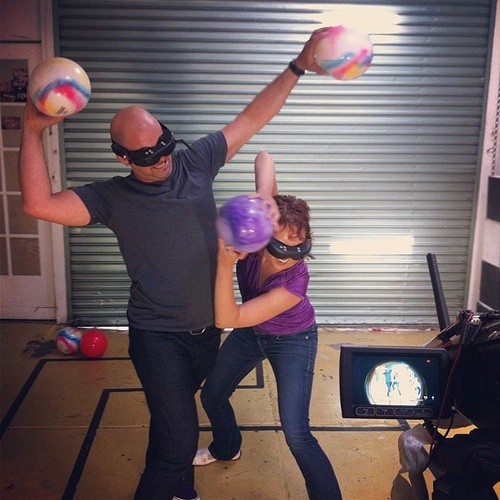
[267,237,312,261]
[133,132,176,169]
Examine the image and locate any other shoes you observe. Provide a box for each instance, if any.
[173,490,200,500]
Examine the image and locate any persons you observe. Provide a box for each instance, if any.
[17,26,335,500]
[191,151,346,500]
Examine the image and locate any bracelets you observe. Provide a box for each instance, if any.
[289,60,305,74]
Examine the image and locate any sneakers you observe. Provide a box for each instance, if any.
[191,445,242,466]
